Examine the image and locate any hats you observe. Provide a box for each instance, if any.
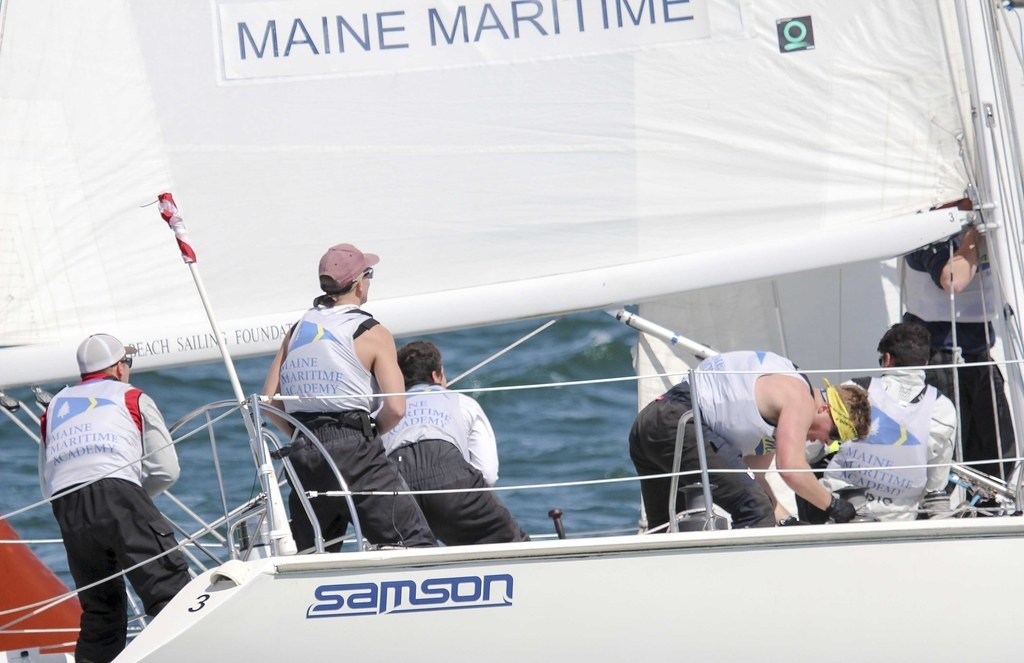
[319,243,379,292]
[76,332,137,374]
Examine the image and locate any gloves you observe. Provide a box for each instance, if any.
[824,496,855,523]
[784,516,805,525]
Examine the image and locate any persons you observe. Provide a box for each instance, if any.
[629,349,869,534]
[36,333,191,663]
[371,341,531,545]
[793,199,1016,525]
[261,242,437,548]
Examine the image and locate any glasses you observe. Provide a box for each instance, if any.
[111,356,132,368]
[878,356,886,366]
[352,267,374,282]
[828,410,841,442]
